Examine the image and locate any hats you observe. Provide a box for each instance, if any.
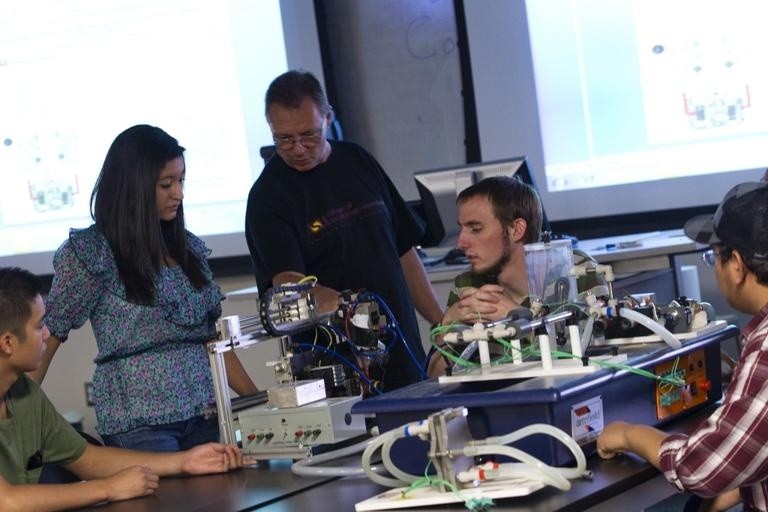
[684,181,767,247]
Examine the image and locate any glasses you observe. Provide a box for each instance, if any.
[273,116,325,150]
[702,247,727,267]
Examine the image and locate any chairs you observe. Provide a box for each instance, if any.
[37,430,103,486]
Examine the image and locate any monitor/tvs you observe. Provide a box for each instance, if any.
[413,156,553,264]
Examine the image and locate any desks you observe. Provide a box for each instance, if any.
[250,386,724,512]
[221,229,719,344]
[71,433,383,511]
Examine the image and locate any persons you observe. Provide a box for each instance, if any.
[245,72,444,402]
[0,262,259,512]
[25,125,259,452]
[596,181,768,511]
[425,176,612,380]
